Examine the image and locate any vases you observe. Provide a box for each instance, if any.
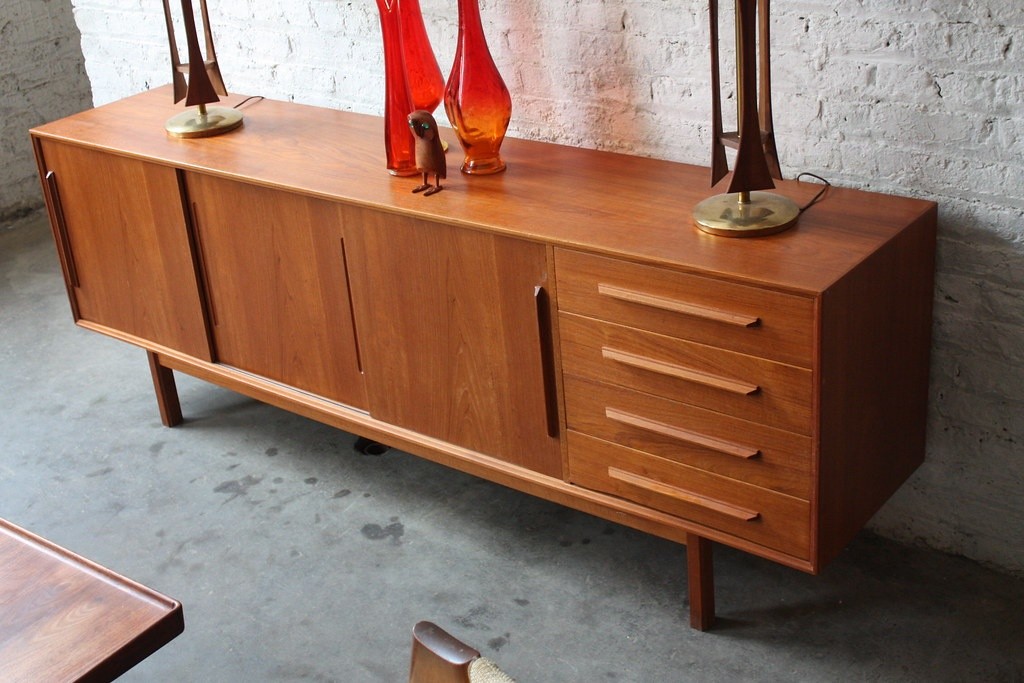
[445,0,512,175]
[399,0,448,151]
[375,0,420,176]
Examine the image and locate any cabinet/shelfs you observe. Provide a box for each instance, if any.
[28,85,940,634]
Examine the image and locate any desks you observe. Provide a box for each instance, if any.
[0,517,185,683]
